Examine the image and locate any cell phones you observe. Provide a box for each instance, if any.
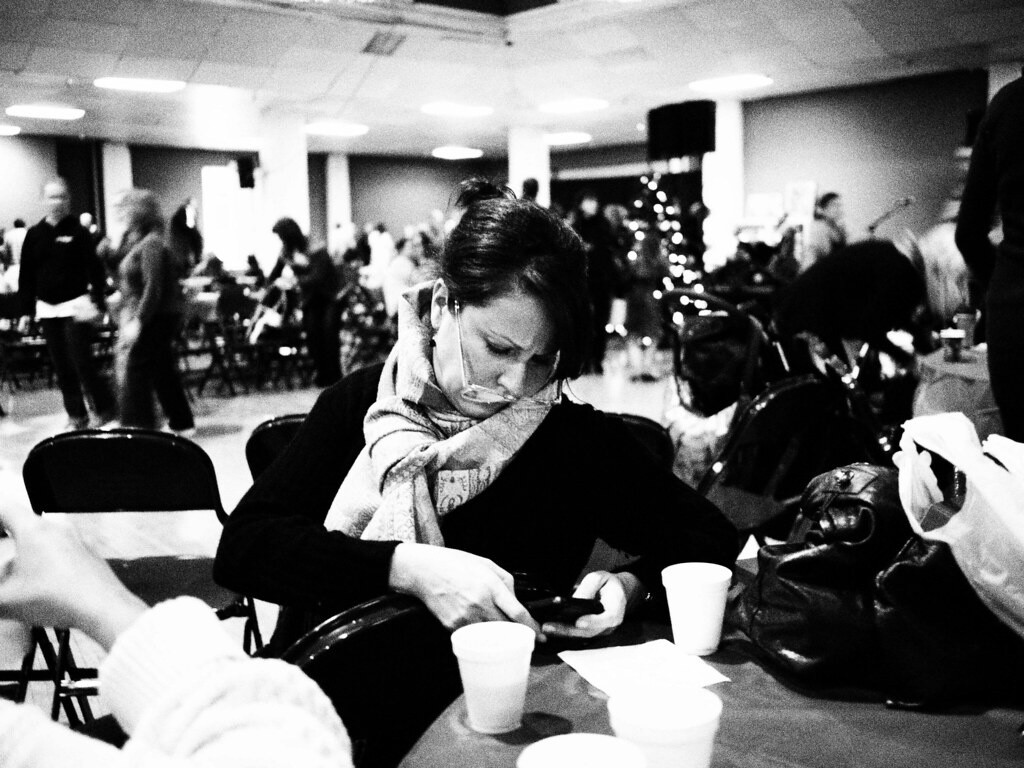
[523,596,604,629]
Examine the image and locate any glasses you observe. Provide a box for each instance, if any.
[453,299,562,410]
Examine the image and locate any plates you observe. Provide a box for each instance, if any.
[516,734,617,768]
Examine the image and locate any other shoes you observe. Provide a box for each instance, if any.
[161,425,196,437]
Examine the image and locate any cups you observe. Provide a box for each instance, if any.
[608,688,723,768]
[955,304,976,347]
[451,621,536,734]
[940,328,966,363]
[663,562,733,657]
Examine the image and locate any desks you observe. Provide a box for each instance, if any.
[402,654,1023,768]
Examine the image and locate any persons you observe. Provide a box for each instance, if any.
[0,213,120,360]
[187,253,304,389]
[209,177,745,767]
[170,194,204,267]
[0,465,356,768]
[807,190,843,263]
[519,176,667,386]
[109,190,199,437]
[953,60,1024,442]
[340,210,459,329]
[13,179,120,431]
[262,215,342,391]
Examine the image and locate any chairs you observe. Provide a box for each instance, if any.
[0,428,463,768]
[693,373,833,497]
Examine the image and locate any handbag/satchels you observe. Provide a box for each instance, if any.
[720,462,997,711]
[892,410,1024,636]
